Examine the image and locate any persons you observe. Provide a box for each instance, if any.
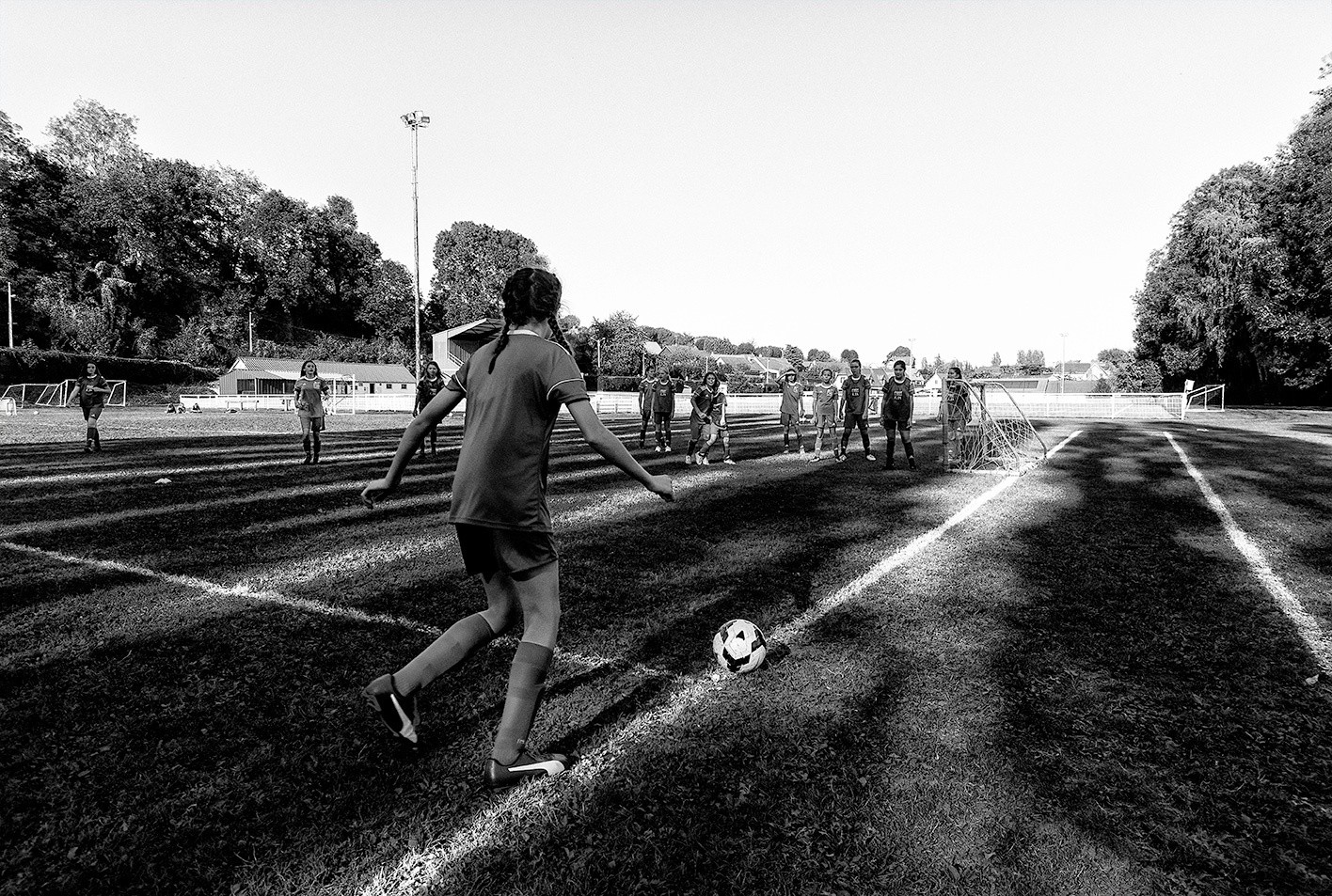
[178,403,185,414]
[685,372,717,465]
[294,360,330,466]
[413,361,445,459]
[810,368,842,463]
[65,361,111,452]
[361,267,674,788]
[840,360,876,460]
[168,402,176,413]
[695,379,736,465]
[879,360,917,472]
[935,367,972,463]
[638,366,666,449]
[191,403,202,413]
[775,368,805,454]
[651,368,674,452]
[676,378,684,393]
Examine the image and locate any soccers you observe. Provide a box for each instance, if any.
[712,618,769,676]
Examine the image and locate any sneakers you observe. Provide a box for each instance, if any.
[365,673,421,752]
[695,452,704,465]
[664,446,671,452]
[702,457,709,465]
[685,455,692,465]
[654,446,662,452]
[724,458,737,465]
[484,745,570,786]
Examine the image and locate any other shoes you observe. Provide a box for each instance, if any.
[84,446,93,452]
[836,457,843,463]
[810,456,820,462]
[909,462,917,470]
[784,449,790,454]
[867,454,876,461]
[305,456,312,464]
[887,460,894,468]
[839,454,847,460]
[799,448,805,454]
